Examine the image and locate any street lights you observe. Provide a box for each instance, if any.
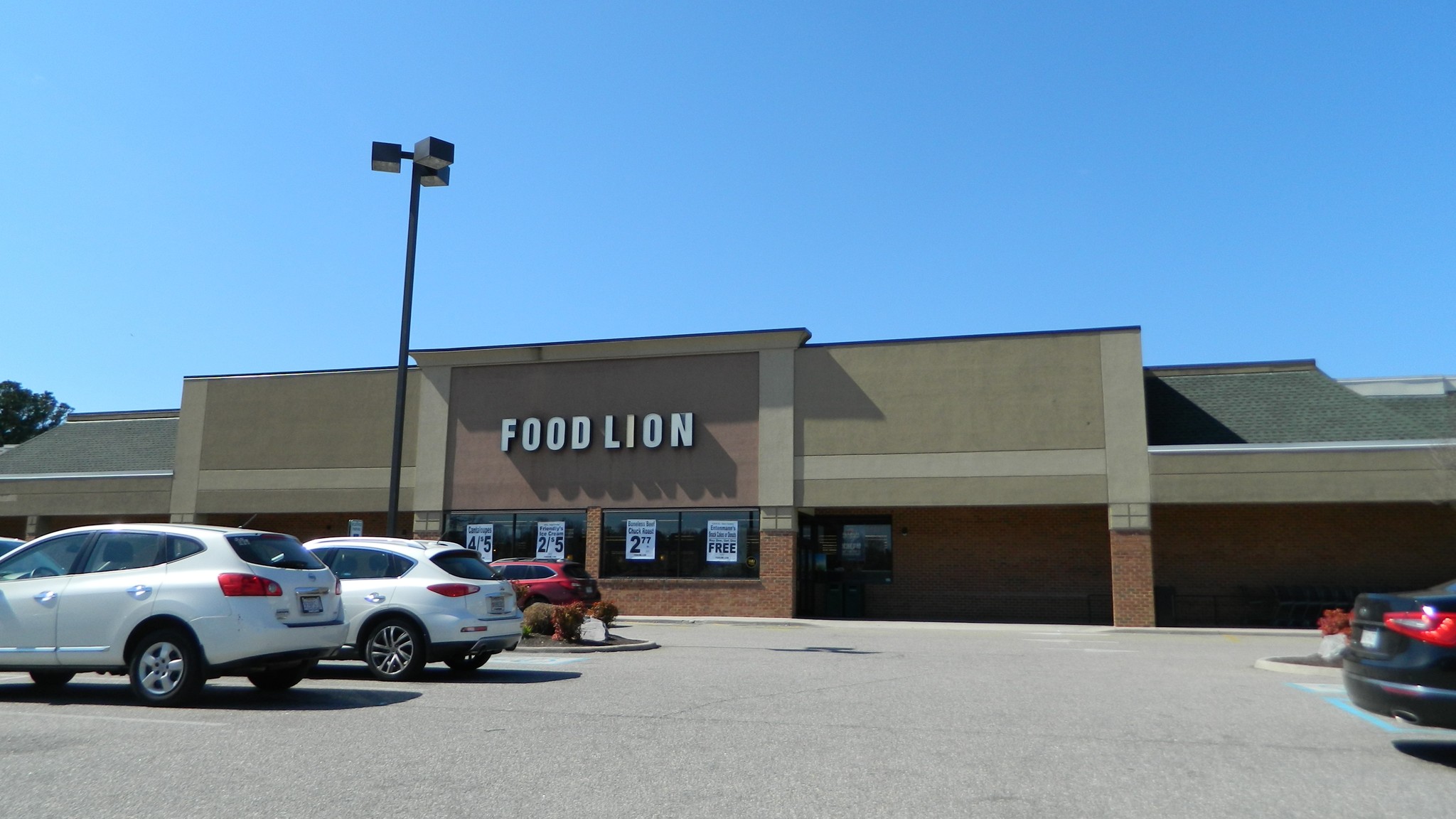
[372,137,455,537]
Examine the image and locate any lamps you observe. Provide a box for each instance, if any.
[902,527,907,536]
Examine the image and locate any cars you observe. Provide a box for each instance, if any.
[0,537,68,581]
[1343,577,1456,729]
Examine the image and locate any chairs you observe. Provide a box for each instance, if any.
[332,555,361,580]
[360,554,388,580]
[98,541,137,570]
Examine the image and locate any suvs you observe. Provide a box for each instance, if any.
[272,536,524,682]
[0,522,350,707]
[490,557,602,611]
